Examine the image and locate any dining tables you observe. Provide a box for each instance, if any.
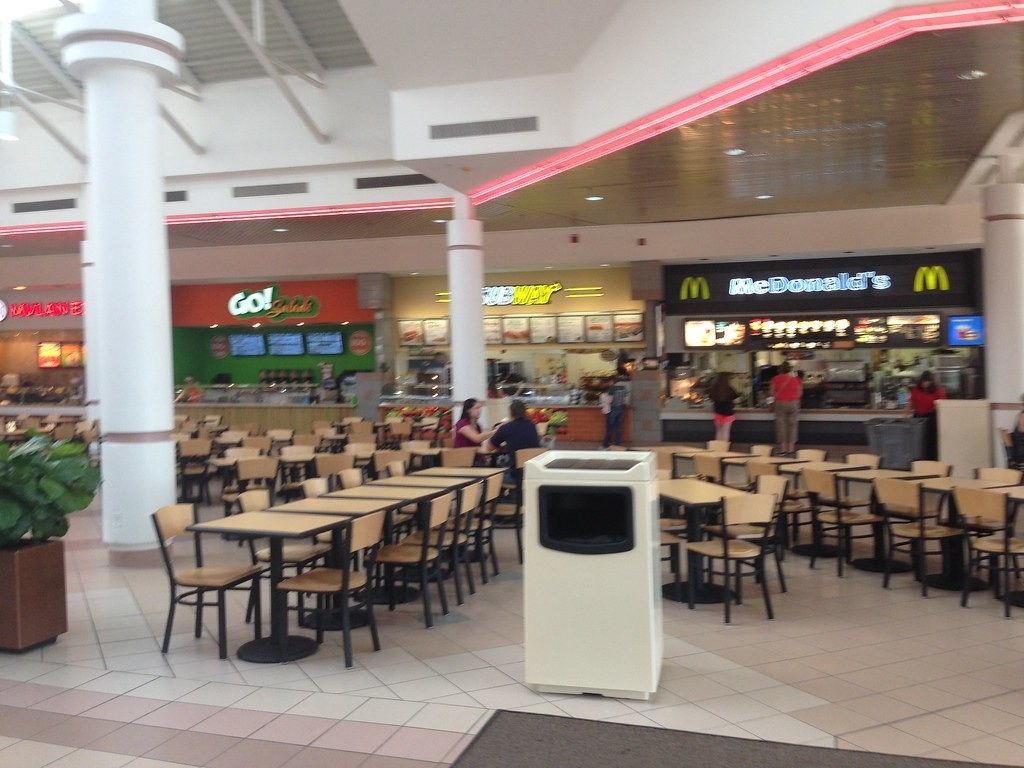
[630,447,1024,609]
[454,445,523,527]
[179,466,512,664]
[0,411,444,506]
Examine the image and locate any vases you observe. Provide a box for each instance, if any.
[385,420,557,435]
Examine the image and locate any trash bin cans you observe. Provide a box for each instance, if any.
[865,416,928,471]
[515,449,663,699]
[933,399,992,469]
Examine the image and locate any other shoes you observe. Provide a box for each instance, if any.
[780,452,795,456]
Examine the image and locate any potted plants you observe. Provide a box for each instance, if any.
[0,431,103,651]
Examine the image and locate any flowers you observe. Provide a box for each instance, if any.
[389,404,569,427]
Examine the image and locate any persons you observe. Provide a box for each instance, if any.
[1012,392,1024,467]
[904,370,947,461]
[174,376,206,403]
[709,372,740,441]
[487,399,539,485]
[598,367,631,450]
[770,361,802,459]
[451,398,506,467]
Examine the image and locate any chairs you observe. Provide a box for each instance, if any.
[151,464,504,670]
[439,422,550,563]
[608,440,882,625]
[871,458,1024,618]
